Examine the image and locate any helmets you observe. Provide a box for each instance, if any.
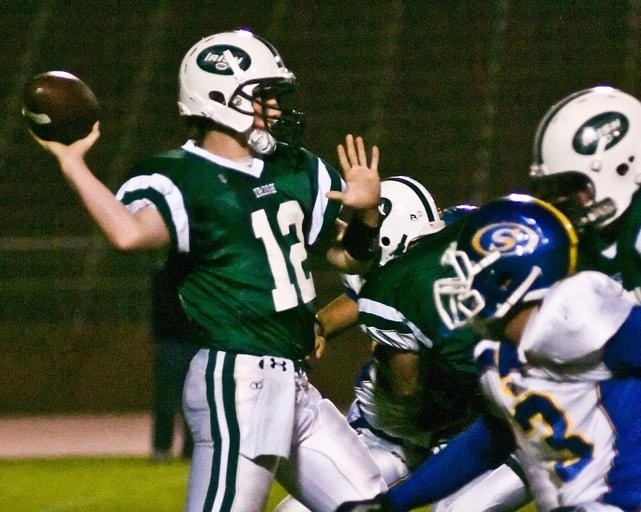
[457,195,577,322]
[438,203,479,226]
[528,87,641,231]
[177,30,304,153]
[378,177,446,266]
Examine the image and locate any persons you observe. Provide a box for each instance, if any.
[24,28,390,512]
[148,247,195,459]
[273,86,641,512]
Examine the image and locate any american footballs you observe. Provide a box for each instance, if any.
[22,71,101,145]
